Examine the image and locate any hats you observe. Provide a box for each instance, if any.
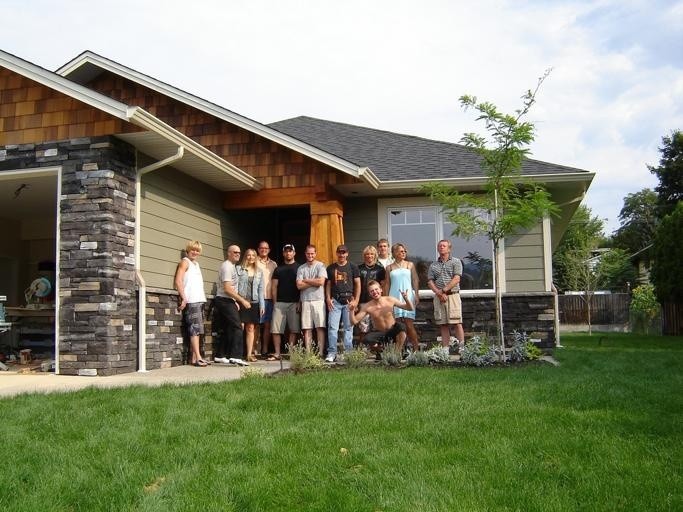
[283,244,295,251]
[337,245,348,253]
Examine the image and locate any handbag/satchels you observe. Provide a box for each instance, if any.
[333,292,353,304]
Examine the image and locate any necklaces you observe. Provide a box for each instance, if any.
[394,260,405,268]
[364,263,375,269]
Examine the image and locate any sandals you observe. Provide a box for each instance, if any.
[266,354,282,360]
[196,359,210,366]
[247,356,257,362]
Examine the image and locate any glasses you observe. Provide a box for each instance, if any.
[400,250,407,254]
[234,251,241,254]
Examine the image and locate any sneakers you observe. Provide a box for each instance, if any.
[230,358,250,366]
[214,356,230,363]
[325,352,337,362]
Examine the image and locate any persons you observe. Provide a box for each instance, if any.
[384,243,419,351]
[235,249,265,361]
[296,244,328,360]
[256,241,278,359]
[213,245,251,366]
[428,240,464,355]
[265,244,302,361]
[347,281,415,365]
[324,245,361,362]
[358,245,391,349]
[377,238,395,270]
[175,240,211,367]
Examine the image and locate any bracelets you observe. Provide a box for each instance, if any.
[442,288,446,294]
